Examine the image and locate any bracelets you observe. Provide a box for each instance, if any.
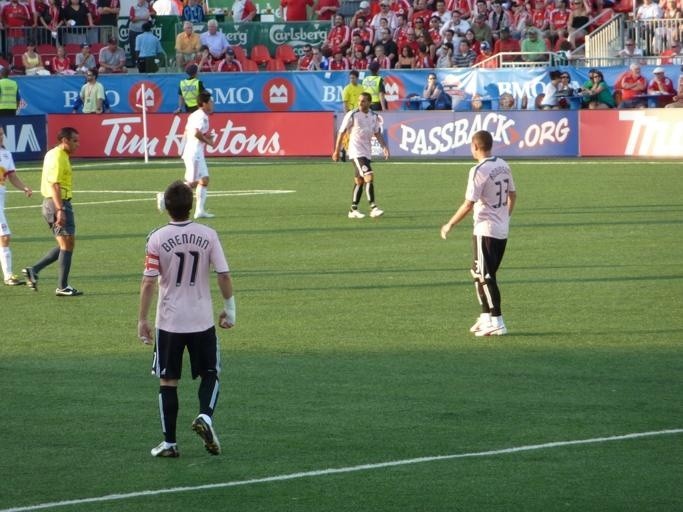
[177,107,182,112]
[55,206,68,212]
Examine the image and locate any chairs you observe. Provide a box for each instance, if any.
[9,43,99,73]
[229,44,298,73]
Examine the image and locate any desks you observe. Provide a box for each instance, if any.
[383,91,673,111]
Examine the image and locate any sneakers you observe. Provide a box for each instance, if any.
[192,416,220,455]
[55,285,82,295]
[21,267,37,291]
[370,207,383,218]
[156,193,166,211]
[150,440,178,456]
[469,318,506,336]
[3,275,26,285]
[347,210,365,219]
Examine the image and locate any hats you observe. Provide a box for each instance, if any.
[79,42,91,48]
[359,1,369,9]
[480,41,491,50]
[527,27,536,34]
[108,37,116,44]
[500,26,508,32]
[379,1,388,6]
[652,67,664,74]
[226,49,233,55]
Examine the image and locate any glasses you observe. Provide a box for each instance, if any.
[575,3,580,5]
[590,75,600,79]
[381,5,385,7]
[559,76,569,79]
[626,45,631,46]
[407,21,437,38]
[672,46,676,48]
[303,50,309,52]
[429,78,435,80]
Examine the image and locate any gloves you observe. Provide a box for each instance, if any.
[223,296,235,325]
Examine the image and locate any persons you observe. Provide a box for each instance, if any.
[339,71,367,163]
[362,63,386,111]
[158,94,217,217]
[71,66,111,115]
[281,0,683,70]
[440,131,515,339]
[21,127,83,297]
[0,69,21,116]
[422,72,448,109]
[0,0,257,76]
[134,180,237,457]
[173,64,205,115]
[542,63,682,110]
[0,125,31,288]
[329,94,389,220]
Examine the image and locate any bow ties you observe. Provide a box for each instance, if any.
[193,211,214,219]
